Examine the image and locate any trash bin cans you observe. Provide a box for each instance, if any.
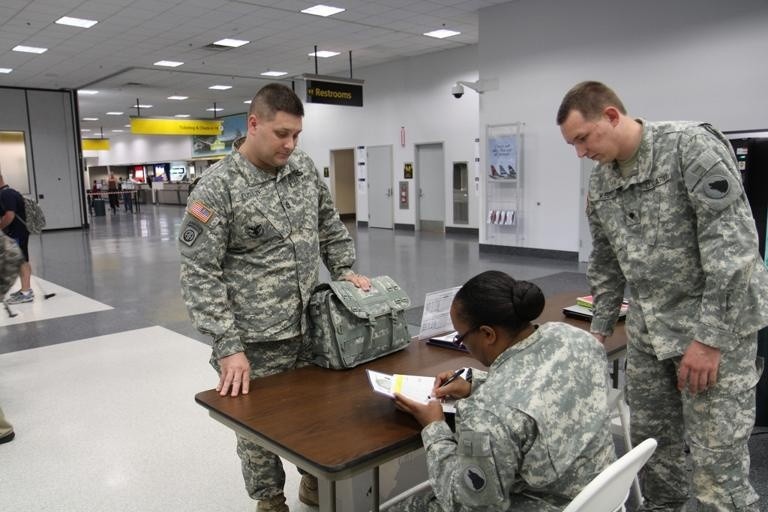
[93,199,106,217]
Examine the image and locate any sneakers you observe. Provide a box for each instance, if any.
[6,291,33,304]
[8,289,34,298]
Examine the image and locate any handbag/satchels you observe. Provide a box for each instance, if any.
[310,278,413,371]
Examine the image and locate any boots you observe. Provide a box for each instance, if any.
[299,474,319,505]
[256,492,290,511]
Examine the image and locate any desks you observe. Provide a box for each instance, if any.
[195,270,651,512]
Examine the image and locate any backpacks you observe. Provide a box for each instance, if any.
[1,186,46,235]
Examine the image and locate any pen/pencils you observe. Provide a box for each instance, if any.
[425,368,465,401]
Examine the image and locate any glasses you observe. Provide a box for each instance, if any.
[452,325,479,348]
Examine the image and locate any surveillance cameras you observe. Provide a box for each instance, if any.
[449,83,466,100]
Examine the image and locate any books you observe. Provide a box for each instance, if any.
[365,354,460,414]
[426,319,473,353]
[562,295,632,323]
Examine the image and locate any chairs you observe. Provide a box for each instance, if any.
[561,437,657,512]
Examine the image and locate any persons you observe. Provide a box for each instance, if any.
[0,407,15,444]
[108,175,133,217]
[178,84,373,512]
[558,80,768,512]
[91,180,100,199]
[1,177,32,305]
[385,262,612,512]
